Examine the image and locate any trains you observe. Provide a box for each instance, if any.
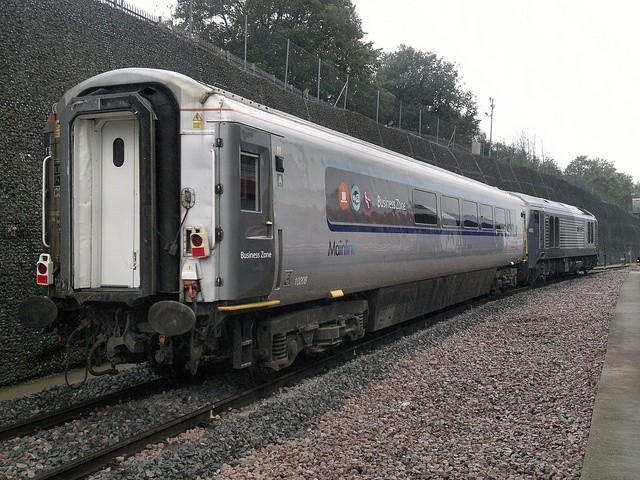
[19,66,599,389]
